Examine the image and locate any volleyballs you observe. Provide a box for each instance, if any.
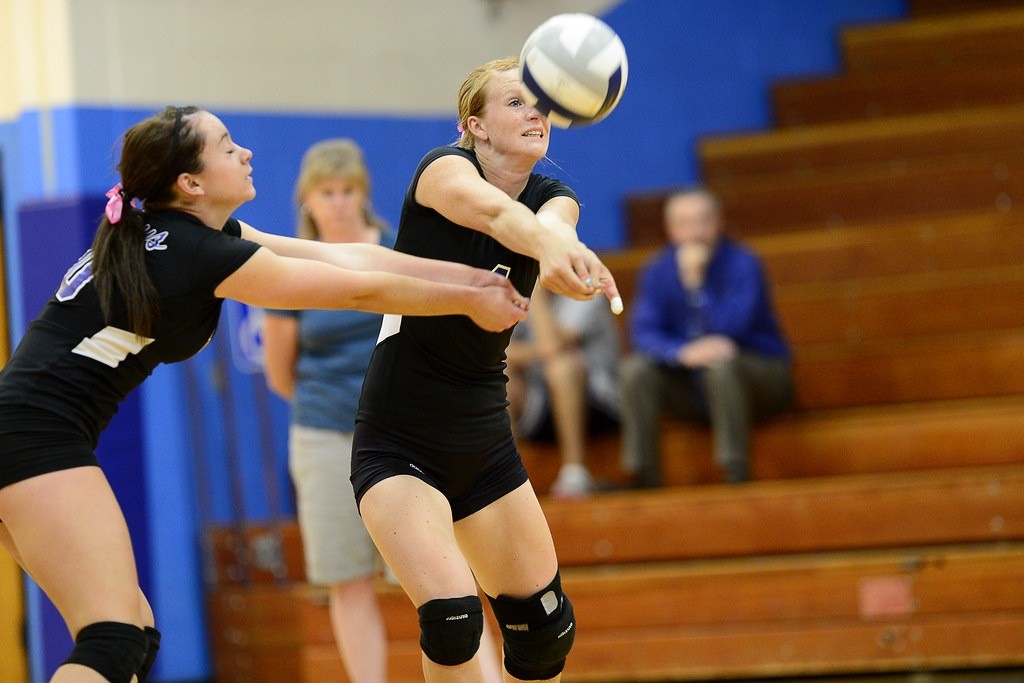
[515,11,631,133]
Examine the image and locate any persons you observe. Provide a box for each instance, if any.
[258,136,502,683]
[351,52,625,683]
[597,183,796,494]
[501,266,637,499]
[0,104,531,683]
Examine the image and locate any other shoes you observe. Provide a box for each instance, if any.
[550,463,593,495]
[598,469,657,493]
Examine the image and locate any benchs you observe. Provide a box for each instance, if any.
[200,2,1023,683]
